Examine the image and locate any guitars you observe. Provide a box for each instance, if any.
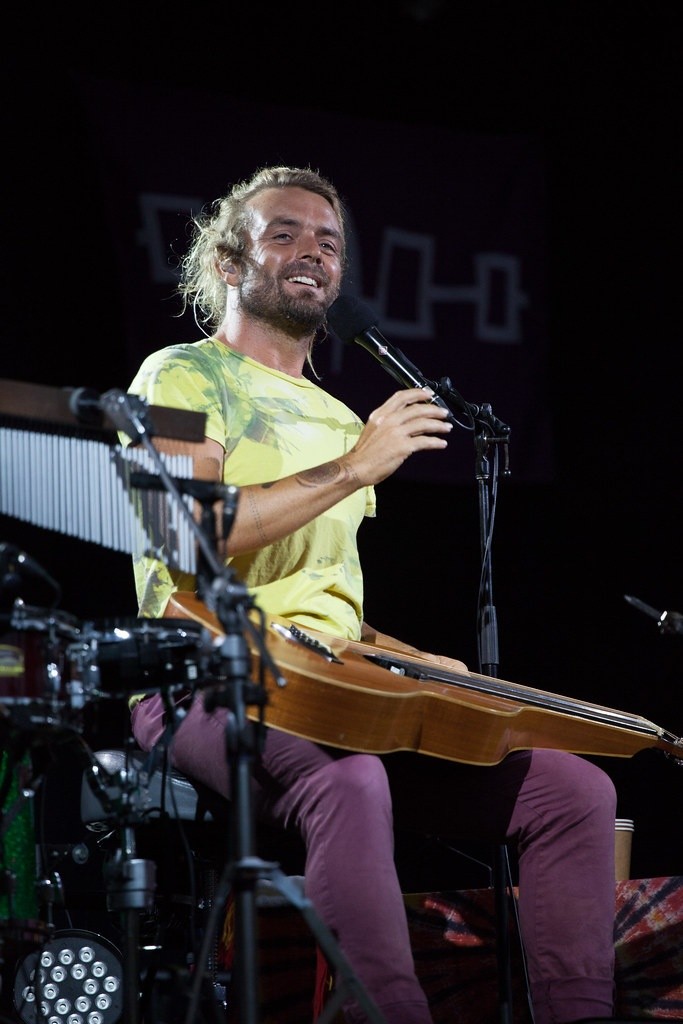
[165,591,681,764]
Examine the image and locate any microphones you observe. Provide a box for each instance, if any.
[69,388,148,418]
[354,325,455,421]
[626,596,683,632]
[130,470,233,504]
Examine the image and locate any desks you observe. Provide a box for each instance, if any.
[211,875,683,1023]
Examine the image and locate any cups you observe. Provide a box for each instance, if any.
[614,819,635,880]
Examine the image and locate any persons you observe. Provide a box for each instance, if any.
[122,166,618,1024]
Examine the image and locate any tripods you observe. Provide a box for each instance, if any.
[116,413,386,1024]
